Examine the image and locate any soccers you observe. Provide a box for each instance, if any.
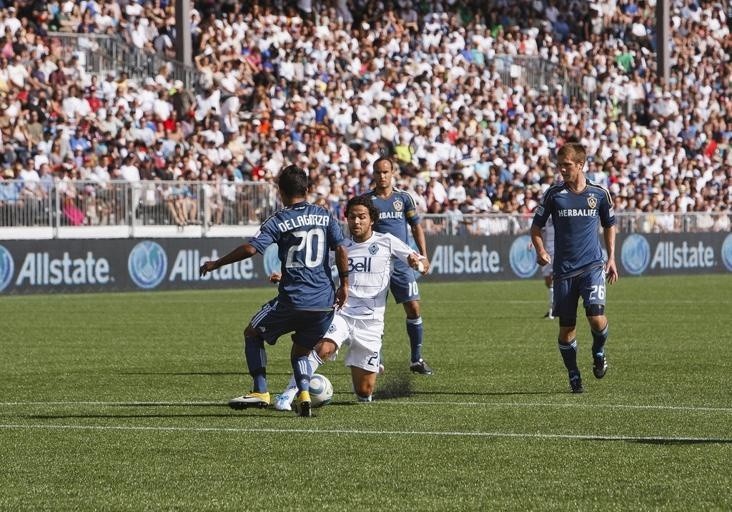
[296,373,334,408]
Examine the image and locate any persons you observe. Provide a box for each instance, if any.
[199,163,351,418]
[529,142,622,394]
[362,156,434,377]
[269,194,431,411]
[1,1,732,236]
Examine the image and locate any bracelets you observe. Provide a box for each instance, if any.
[414,261,425,274]
[338,270,349,279]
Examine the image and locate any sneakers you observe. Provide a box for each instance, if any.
[409,358,432,375]
[228,390,271,409]
[591,344,608,378]
[273,393,292,411]
[293,390,312,417]
[568,370,584,393]
